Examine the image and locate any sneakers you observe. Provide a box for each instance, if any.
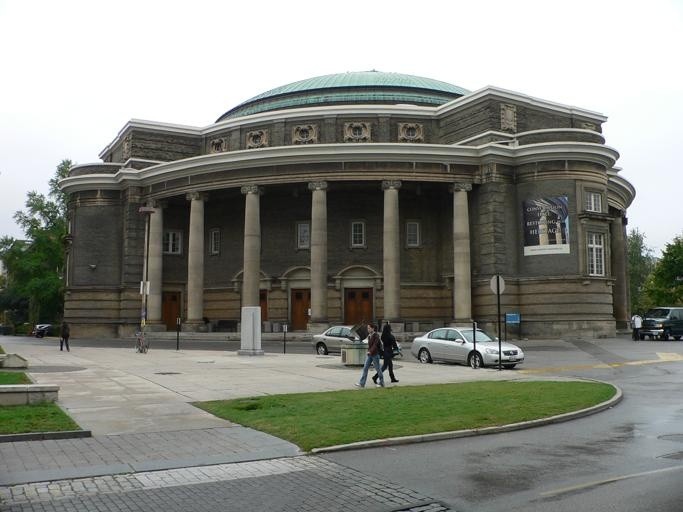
[376,385,384,388]
[372,376,378,384]
[352,383,364,390]
[391,379,399,383]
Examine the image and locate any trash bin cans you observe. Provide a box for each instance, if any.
[341,344,369,366]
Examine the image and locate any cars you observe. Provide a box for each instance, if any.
[29,323,57,339]
[309,323,403,359]
[408,324,528,367]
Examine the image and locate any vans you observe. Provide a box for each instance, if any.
[630,304,682,342]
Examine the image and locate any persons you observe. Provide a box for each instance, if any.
[352,323,384,389]
[59,321,70,352]
[631,312,644,341]
[372,324,403,384]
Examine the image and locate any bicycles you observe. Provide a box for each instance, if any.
[130,323,153,353]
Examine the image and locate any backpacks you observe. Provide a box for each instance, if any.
[374,334,384,354]
